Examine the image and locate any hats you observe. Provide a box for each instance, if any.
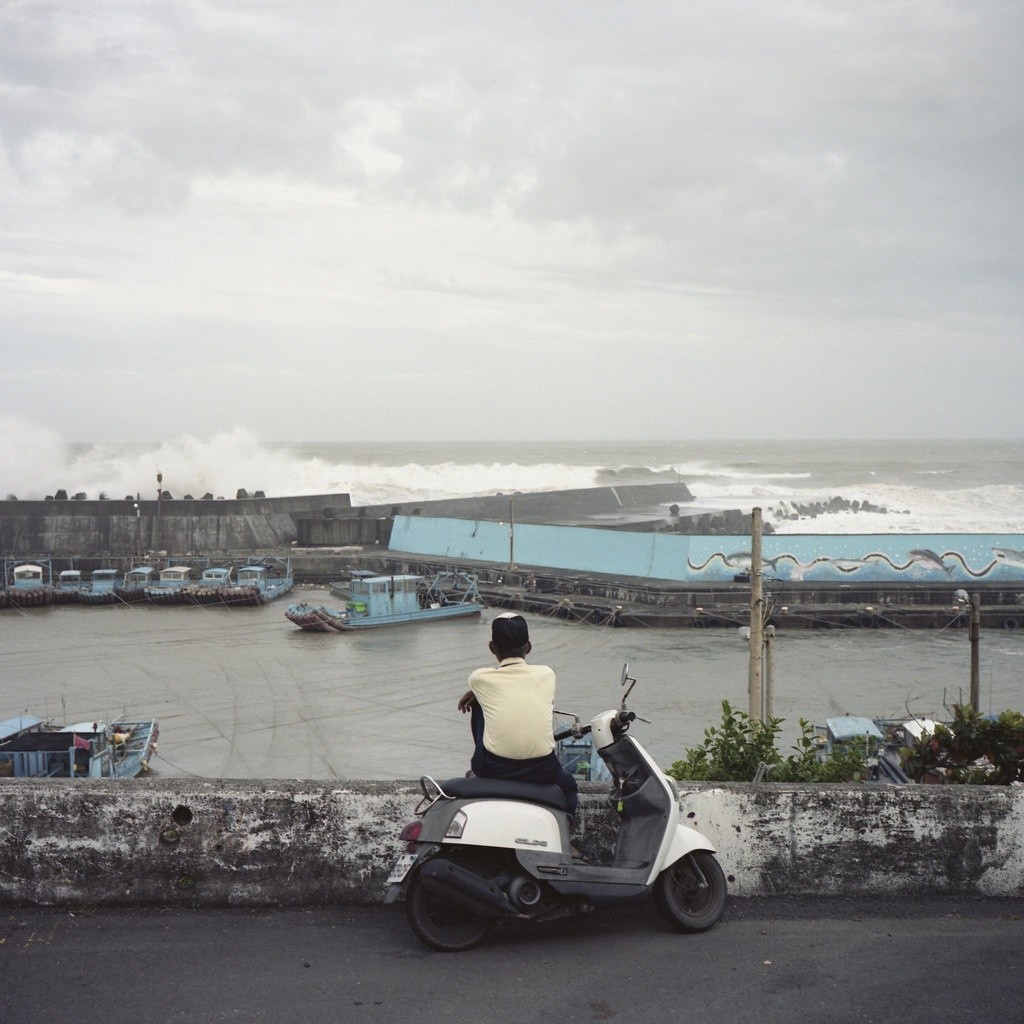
[491,611,530,649]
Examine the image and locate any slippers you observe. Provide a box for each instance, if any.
[571,851,592,867]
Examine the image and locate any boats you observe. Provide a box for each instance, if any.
[284,570,483,633]
[91,712,160,776]
[6,565,53,606]
[81,570,118,605]
[116,566,294,607]
[812,715,960,783]
[51,570,86,601]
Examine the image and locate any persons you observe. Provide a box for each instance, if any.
[457,613,590,862]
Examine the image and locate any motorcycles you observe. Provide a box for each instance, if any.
[385,660,728,951]
